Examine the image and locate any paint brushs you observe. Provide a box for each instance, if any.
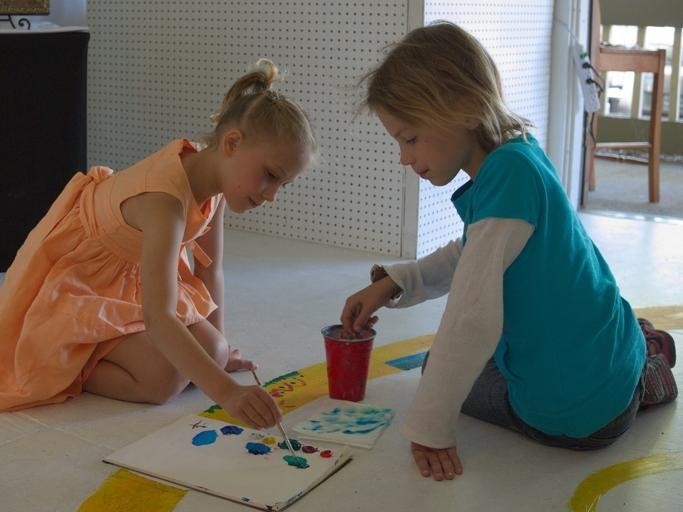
[353,304,361,339]
[250,368,310,468]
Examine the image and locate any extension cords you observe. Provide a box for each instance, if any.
[572,45,600,114]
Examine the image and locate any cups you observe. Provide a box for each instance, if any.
[321,324,377,402]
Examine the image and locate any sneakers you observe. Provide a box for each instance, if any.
[643,353,678,406]
[638,317,675,370]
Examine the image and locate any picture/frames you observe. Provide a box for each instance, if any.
[0,0,49,16]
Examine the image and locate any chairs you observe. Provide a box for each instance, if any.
[577,0,665,210]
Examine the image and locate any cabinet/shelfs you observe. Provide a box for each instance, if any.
[0,28,88,274]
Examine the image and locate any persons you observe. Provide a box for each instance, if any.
[337,20,678,483]
[0,56,320,430]
